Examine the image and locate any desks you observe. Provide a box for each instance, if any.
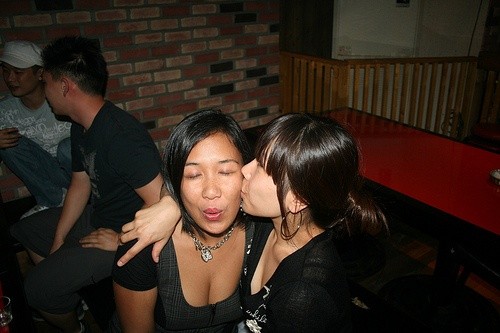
[256,107,500,235]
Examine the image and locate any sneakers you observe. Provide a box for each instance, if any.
[20,188,66,219]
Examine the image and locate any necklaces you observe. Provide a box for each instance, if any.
[188,226,236,263]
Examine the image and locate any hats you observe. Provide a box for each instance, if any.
[0,38,42,69]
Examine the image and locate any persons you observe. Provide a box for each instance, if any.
[117,112,388,333]
[112,108,257,333]
[10,35,163,333]
[0,41,73,221]
